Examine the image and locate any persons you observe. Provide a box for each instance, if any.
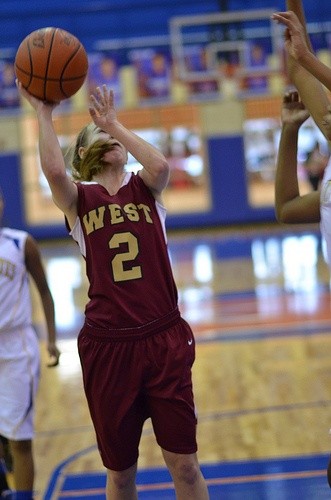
[269,0,331,488]
[15,79,209,500]
[0,44,265,108]
[0,194,61,500]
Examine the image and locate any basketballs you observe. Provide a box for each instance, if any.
[13,26,90,104]
